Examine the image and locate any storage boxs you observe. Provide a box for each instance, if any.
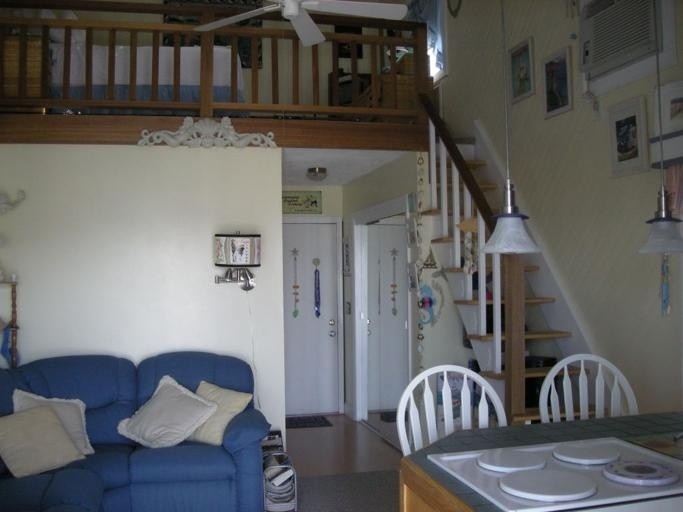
[398,53,430,74]
[380,74,434,109]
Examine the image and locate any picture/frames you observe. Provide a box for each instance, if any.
[542,45,575,120]
[509,35,536,105]
[605,96,650,178]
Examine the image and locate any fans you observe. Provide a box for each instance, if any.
[192,0,409,47]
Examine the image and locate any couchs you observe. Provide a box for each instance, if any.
[0,351,272,512]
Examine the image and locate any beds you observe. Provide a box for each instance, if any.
[50,41,248,118]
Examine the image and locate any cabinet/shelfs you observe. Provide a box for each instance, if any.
[264,452,297,512]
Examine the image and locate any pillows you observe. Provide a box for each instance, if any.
[186,380,254,446]
[60,9,87,44]
[1,406,87,478]
[116,375,218,448]
[39,8,65,41]
[13,388,96,456]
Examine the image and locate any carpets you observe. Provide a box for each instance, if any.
[297,470,399,512]
[286,414,332,430]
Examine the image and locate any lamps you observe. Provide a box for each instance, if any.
[483,1,539,255]
[306,166,328,182]
[638,3,683,254]
[213,231,262,290]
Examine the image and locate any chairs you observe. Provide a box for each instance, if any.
[538,354,639,424]
[396,364,508,457]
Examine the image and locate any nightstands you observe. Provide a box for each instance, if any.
[0,34,50,116]
